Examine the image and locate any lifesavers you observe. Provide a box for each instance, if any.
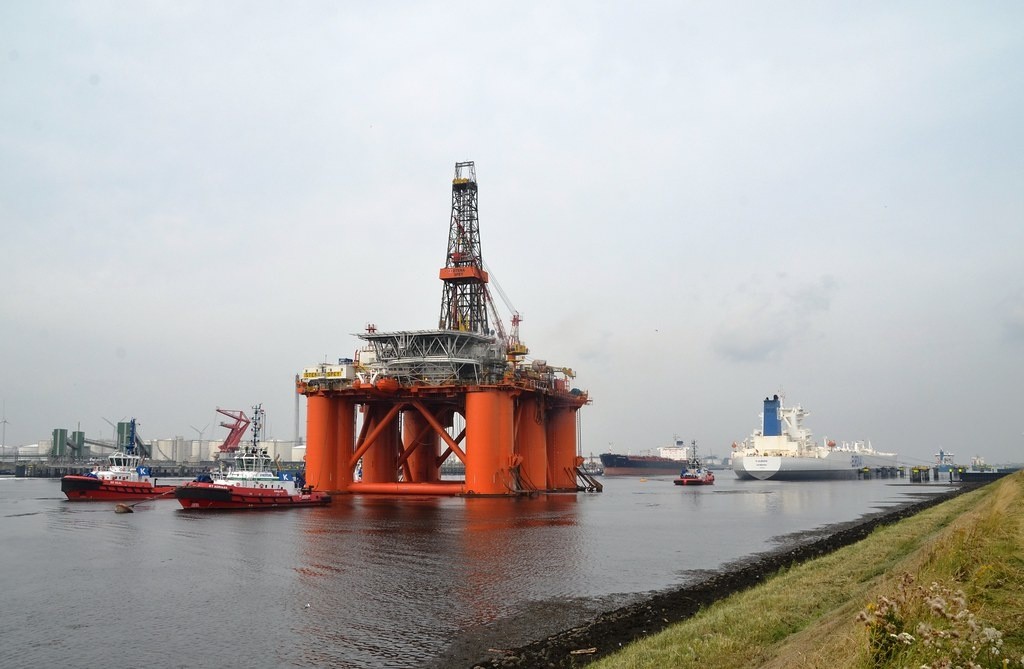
[259,483,264,488]
[236,482,240,486]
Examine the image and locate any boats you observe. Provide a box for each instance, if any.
[673,439,715,485]
[599,453,689,476]
[174,452,332,511]
[61,451,176,502]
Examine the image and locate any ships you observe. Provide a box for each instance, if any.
[731,389,897,481]
